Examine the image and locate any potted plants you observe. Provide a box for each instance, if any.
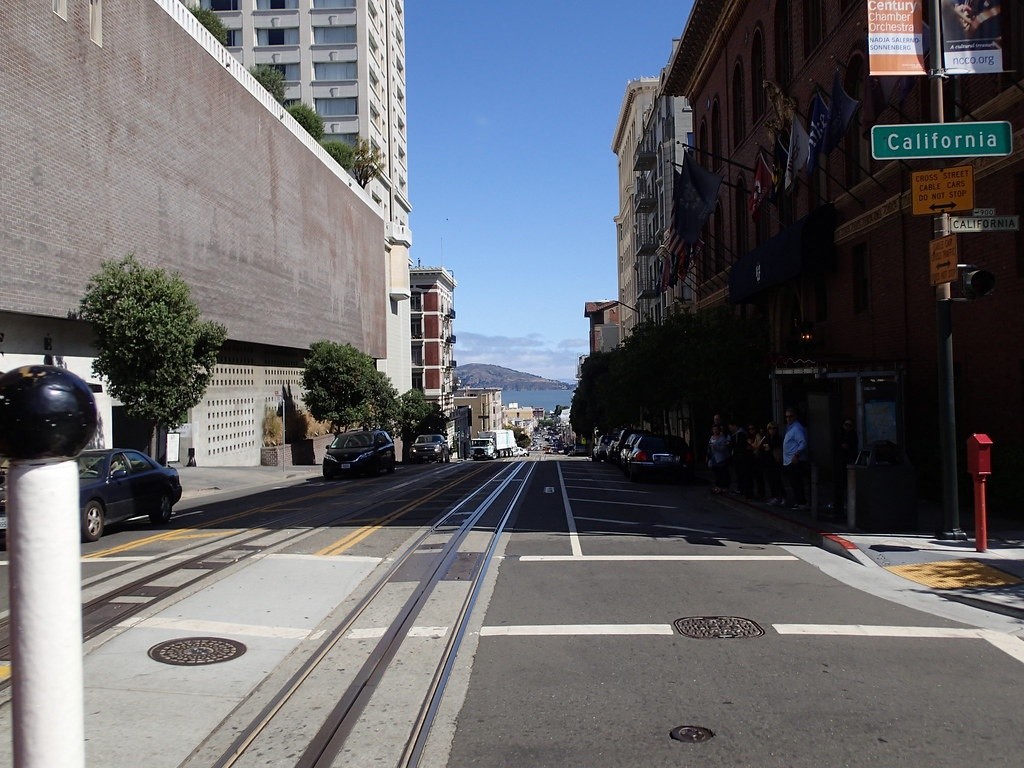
[261,410,292,466]
[299,409,335,466]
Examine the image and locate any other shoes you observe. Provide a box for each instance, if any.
[791,504,809,510]
[766,498,787,507]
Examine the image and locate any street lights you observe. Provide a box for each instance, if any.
[595,301,642,338]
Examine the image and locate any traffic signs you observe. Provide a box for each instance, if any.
[911,165,974,216]
[928,234,959,287]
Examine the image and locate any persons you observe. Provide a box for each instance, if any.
[762,421,786,508]
[728,420,747,496]
[839,419,858,470]
[713,414,729,439]
[744,422,768,500]
[708,424,729,495]
[782,405,808,512]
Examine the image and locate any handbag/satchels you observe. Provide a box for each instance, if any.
[772,447,783,463]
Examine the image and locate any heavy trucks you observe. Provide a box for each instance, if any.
[501,447,529,457]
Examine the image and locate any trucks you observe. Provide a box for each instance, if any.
[469,429,514,461]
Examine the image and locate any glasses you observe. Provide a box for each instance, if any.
[845,423,853,427]
[785,414,794,417]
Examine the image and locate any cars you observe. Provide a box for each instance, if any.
[574,446,588,455]
[322,431,397,480]
[73,449,183,542]
[591,434,613,462]
[623,434,693,483]
[527,426,564,455]
[620,434,641,470]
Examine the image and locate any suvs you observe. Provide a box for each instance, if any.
[410,435,450,464]
[607,428,650,467]
